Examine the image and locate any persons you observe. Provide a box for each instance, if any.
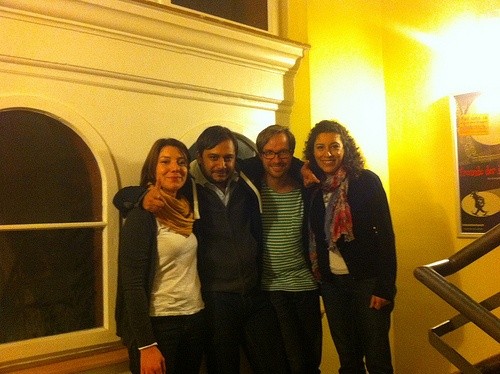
[115,138,205,374]
[112,120,396,374]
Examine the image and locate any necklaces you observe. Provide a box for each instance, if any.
[324,193,330,202]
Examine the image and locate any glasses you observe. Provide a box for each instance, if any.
[262,150,291,160]
